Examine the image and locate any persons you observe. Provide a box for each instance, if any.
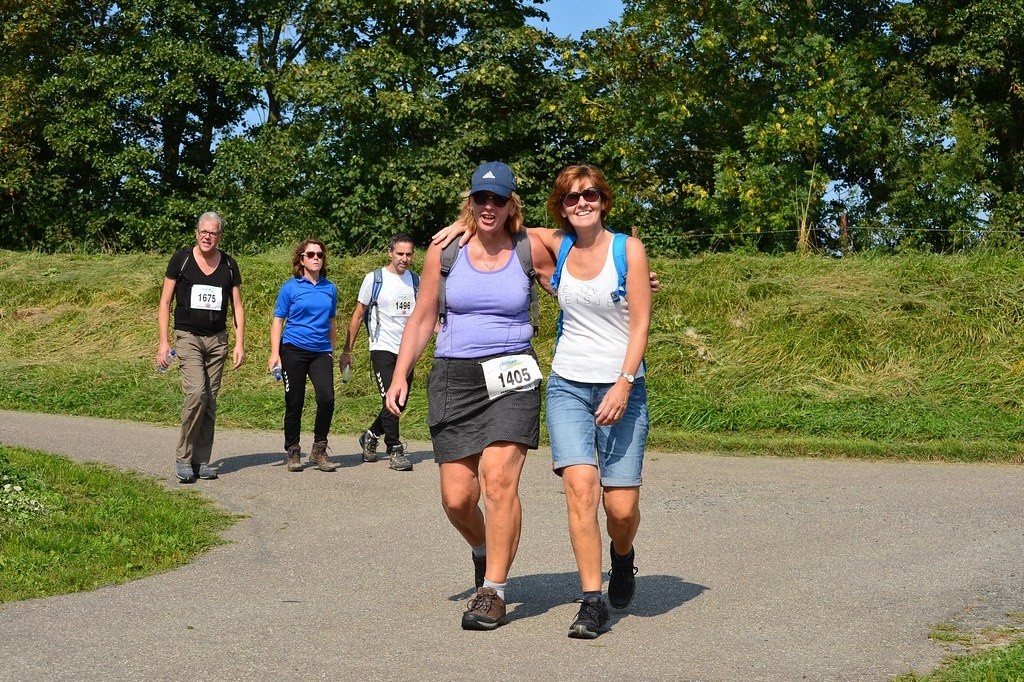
[385,163,660,627]
[432,166,654,638]
[339,234,440,471]
[157,211,245,481]
[268,239,338,472]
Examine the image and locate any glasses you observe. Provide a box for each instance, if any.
[301,252,326,259]
[472,193,512,207]
[198,229,219,238]
[562,187,600,206]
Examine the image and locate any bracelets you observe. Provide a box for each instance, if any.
[343,352,352,355]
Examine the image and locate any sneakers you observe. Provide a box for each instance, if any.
[194,464,219,479]
[608,541,638,609]
[175,462,195,479]
[287,445,306,469]
[567,598,612,636]
[461,587,513,628]
[309,440,337,472]
[388,442,414,469]
[473,551,486,590]
[359,430,381,460]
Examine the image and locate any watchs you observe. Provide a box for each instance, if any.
[620,373,635,384]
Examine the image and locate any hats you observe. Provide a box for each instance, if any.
[468,161,516,196]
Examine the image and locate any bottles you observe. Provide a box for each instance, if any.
[159,350,175,372]
[273,364,282,380]
[341,364,351,383]
